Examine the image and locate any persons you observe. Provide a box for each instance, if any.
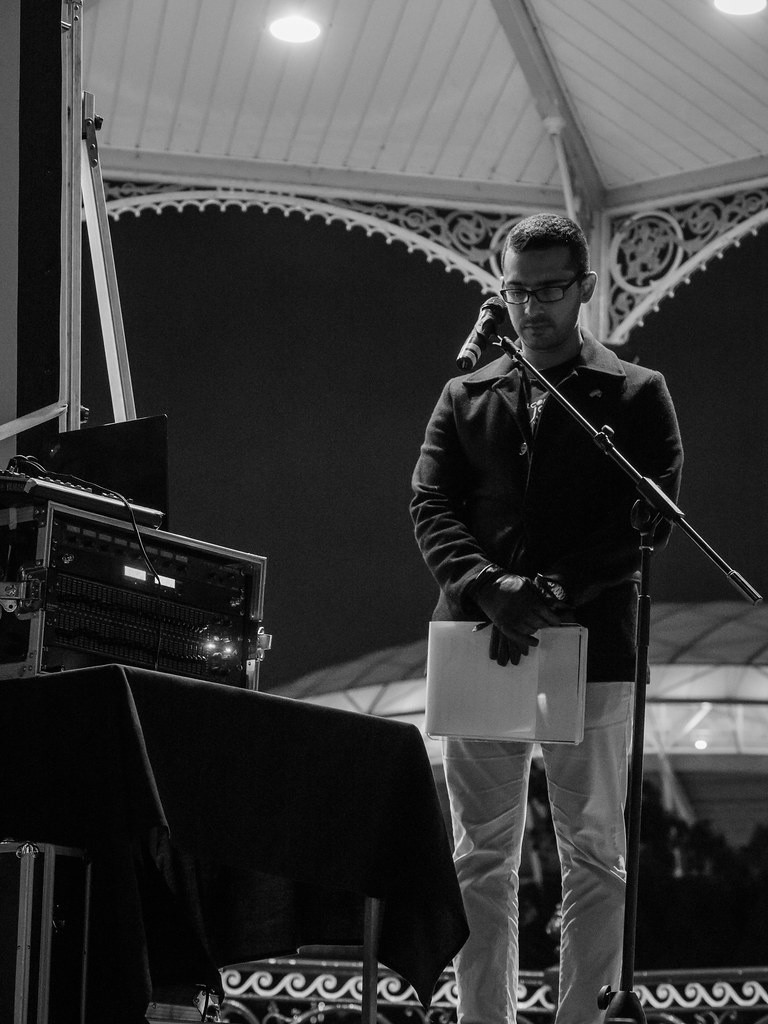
[408,211,683,1024]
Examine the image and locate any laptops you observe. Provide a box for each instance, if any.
[54,412,169,536]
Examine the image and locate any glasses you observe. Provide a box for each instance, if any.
[499,276,578,304]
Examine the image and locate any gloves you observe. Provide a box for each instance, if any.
[487,574,569,665]
[477,574,560,647]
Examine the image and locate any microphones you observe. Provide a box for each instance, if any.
[457,297,507,373]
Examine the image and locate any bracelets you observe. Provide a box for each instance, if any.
[536,574,566,601]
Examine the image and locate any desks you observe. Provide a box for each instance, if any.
[0,663,471,1024]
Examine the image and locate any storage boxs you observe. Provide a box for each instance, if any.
[0,845,96,1024]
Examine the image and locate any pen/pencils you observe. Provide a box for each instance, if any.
[472,619,493,632]
